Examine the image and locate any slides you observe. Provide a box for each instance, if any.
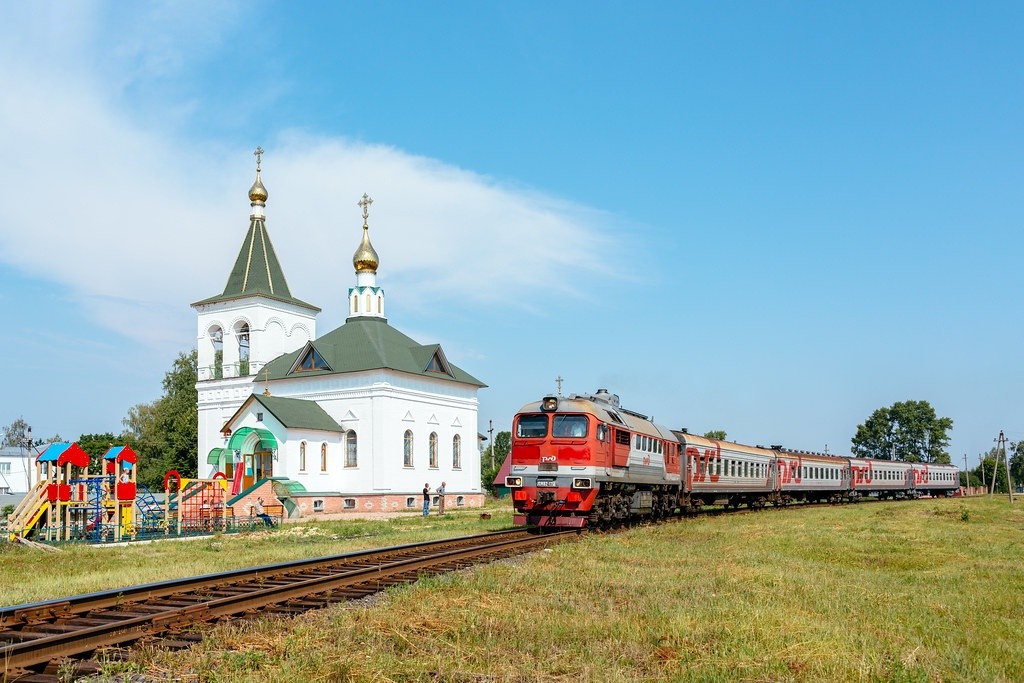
[85,511,115,532]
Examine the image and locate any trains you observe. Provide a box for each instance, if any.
[504,384,960,533]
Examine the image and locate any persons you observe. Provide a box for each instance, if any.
[423,483,431,516]
[198,499,230,531]
[436,482,446,514]
[119,469,135,484]
[570,421,586,437]
[254,497,276,528]
